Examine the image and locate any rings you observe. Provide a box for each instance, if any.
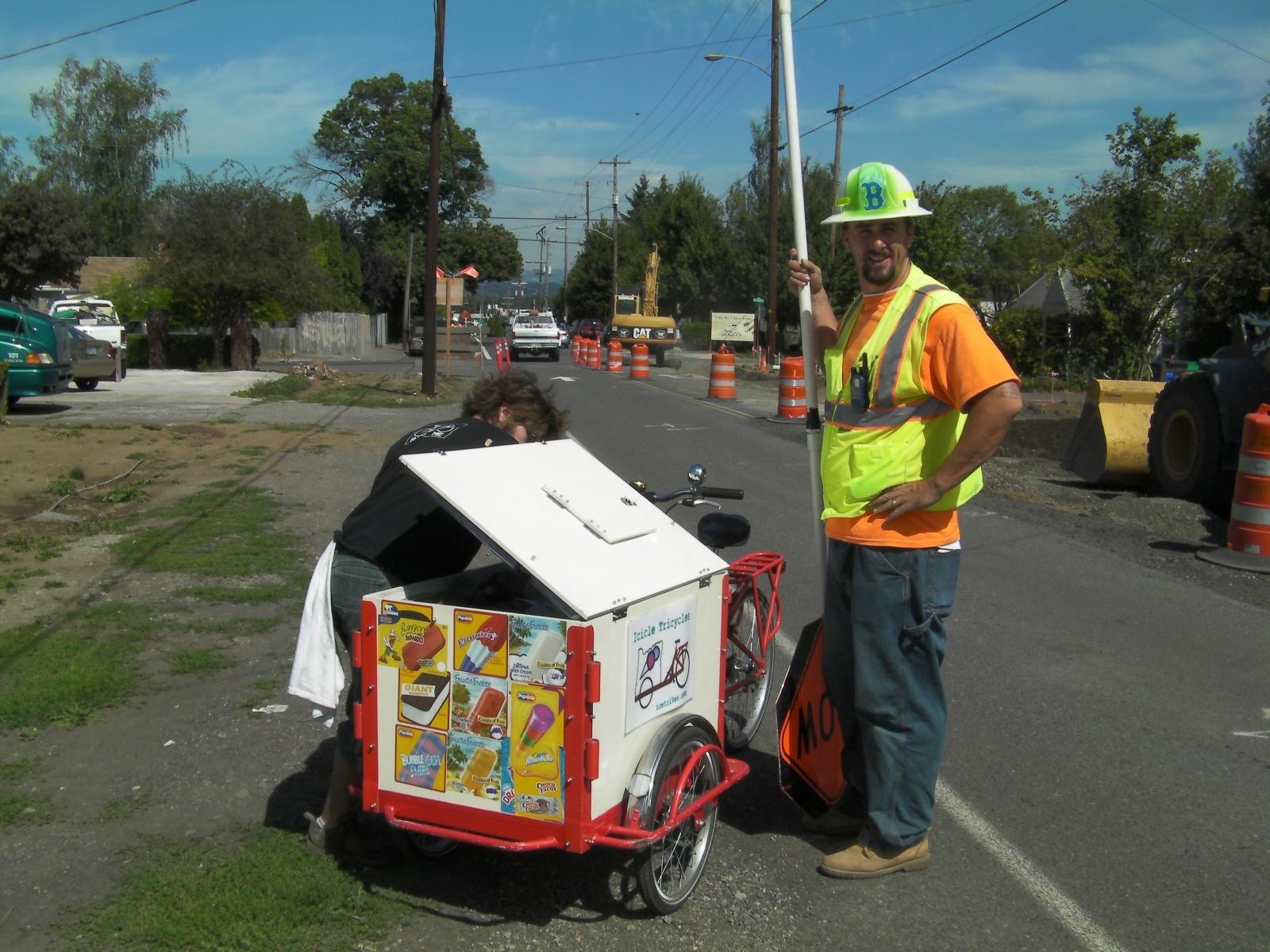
[892,498,896,508]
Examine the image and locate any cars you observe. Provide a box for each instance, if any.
[504,307,605,363]
[0,299,74,410]
[56,318,119,393]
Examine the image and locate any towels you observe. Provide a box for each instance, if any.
[286,539,345,730]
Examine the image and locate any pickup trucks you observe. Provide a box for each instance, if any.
[47,296,128,381]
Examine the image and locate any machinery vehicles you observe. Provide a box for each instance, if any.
[1059,287,1270,514]
[602,238,684,369]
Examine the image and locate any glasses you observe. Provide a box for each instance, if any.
[520,420,541,443]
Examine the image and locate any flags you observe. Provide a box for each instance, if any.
[460,266,479,278]
[436,267,444,278]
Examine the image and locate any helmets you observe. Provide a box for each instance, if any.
[820,162,933,224]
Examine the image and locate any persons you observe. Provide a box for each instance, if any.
[787,162,1023,879]
[302,365,570,865]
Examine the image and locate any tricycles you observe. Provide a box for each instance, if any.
[350,434,789,916]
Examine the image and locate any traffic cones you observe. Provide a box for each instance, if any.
[759,355,768,372]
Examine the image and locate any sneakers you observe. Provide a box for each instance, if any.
[801,806,871,832]
[821,831,929,879]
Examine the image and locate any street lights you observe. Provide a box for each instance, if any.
[555,226,618,296]
[704,53,780,366]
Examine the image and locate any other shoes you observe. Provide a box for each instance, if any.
[305,811,399,866]
